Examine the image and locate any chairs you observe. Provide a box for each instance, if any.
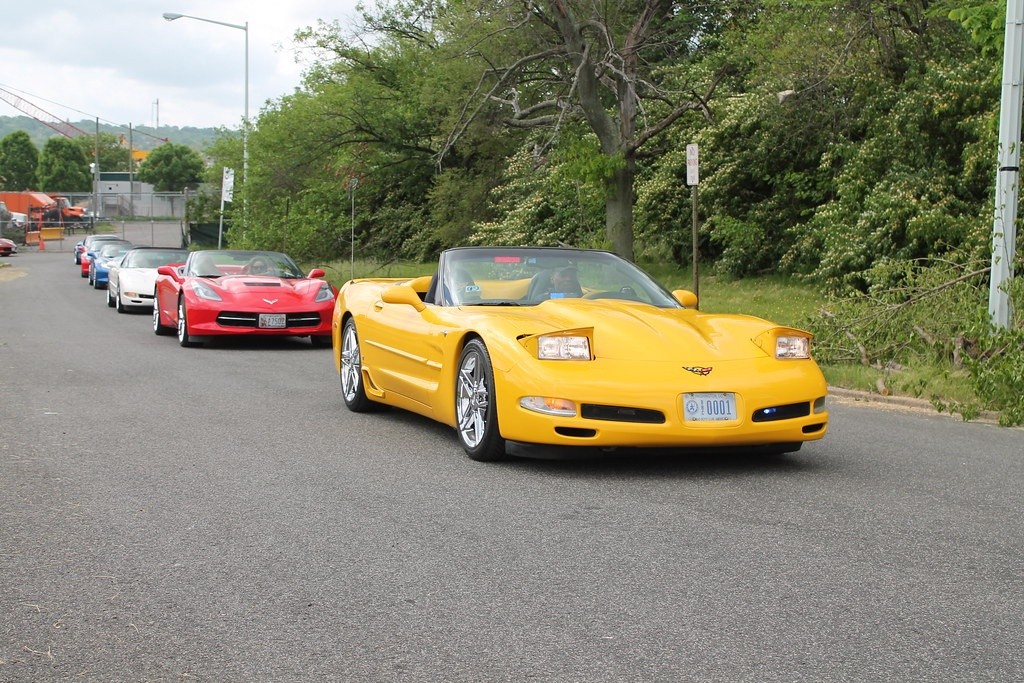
[422,268,480,305]
[525,267,583,303]
[193,257,220,276]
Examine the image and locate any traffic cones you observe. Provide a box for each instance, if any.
[39,236,45,250]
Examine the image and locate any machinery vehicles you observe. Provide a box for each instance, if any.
[0,84,168,162]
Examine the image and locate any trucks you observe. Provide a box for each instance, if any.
[52,197,86,221]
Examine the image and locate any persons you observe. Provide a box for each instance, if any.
[195,254,221,274]
[526,257,583,306]
[247,258,276,277]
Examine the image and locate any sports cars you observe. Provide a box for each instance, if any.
[87,245,131,289]
[78,240,129,278]
[74,235,120,265]
[332,248,829,461]
[153,250,340,348]
[106,247,190,313]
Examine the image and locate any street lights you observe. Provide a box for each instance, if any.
[122,220,125,240]
[151,220,154,246]
[163,12,249,238]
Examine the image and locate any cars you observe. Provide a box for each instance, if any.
[0,238,18,256]
